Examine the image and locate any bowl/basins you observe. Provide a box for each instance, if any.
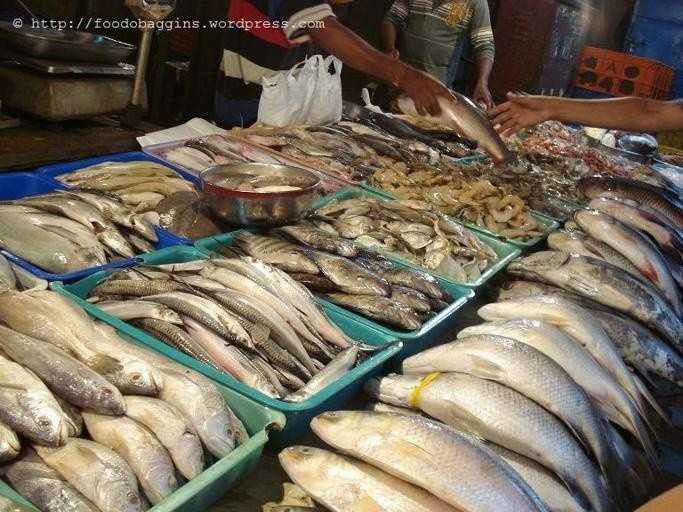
[199,162,322,229]
[581,124,658,164]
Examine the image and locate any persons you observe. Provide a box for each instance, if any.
[212,0,460,130]
[380,0,495,115]
[483,92,683,137]
[301,0,354,63]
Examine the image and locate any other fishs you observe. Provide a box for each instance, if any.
[1,107,683,511]
[395,86,519,173]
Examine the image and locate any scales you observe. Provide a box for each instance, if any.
[0,21,149,123]
